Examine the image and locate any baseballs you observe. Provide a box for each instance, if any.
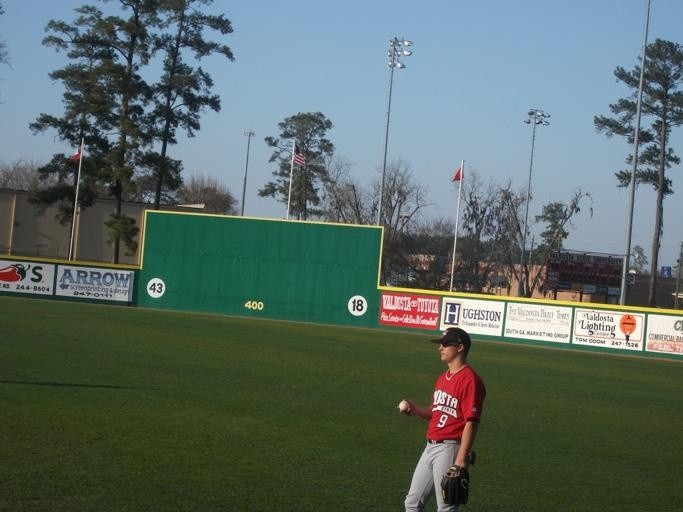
[399,401,410,411]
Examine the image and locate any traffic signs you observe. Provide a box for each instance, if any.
[516,107,553,296]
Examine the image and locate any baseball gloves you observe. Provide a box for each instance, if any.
[441,465,469,506]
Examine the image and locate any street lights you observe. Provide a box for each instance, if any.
[66,45,95,262]
[375,34,416,226]
[239,130,255,217]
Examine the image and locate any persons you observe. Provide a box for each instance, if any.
[394,326,487,512]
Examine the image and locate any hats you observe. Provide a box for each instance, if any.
[430,328,470,345]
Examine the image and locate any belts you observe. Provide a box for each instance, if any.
[429,439,443,444]
